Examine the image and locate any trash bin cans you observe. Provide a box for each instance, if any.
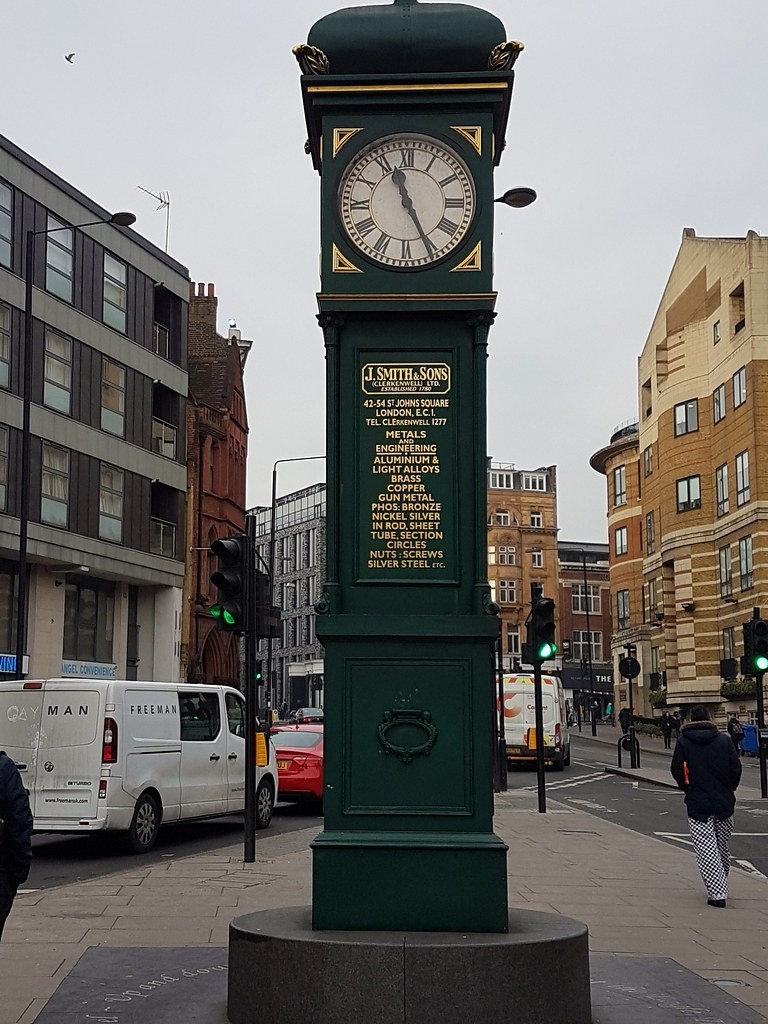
[740,725,757,753]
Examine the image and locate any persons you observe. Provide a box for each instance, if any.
[660,710,682,749]
[280,699,288,720]
[618,708,630,733]
[0,750,33,940]
[671,706,741,908]
[727,715,745,755]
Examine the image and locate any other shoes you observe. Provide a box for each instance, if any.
[707,899,725,907]
[669,747,671,749]
[665,747,668,749]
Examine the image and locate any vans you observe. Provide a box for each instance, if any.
[0,677,279,853]
[495,674,571,771]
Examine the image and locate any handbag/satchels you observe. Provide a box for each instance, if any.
[618,717,621,722]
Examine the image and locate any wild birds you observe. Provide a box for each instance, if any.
[65,53,76,64]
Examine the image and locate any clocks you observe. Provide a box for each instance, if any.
[330,120,484,278]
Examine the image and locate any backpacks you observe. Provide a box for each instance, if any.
[731,722,743,734]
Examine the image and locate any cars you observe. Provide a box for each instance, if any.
[294,708,324,721]
[268,725,324,813]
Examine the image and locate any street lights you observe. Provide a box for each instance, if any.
[16,211,136,680]
[525,547,597,737]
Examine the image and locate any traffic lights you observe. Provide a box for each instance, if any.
[211,534,249,632]
[535,598,557,661]
[256,670,263,685]
[749,619,768,675]
